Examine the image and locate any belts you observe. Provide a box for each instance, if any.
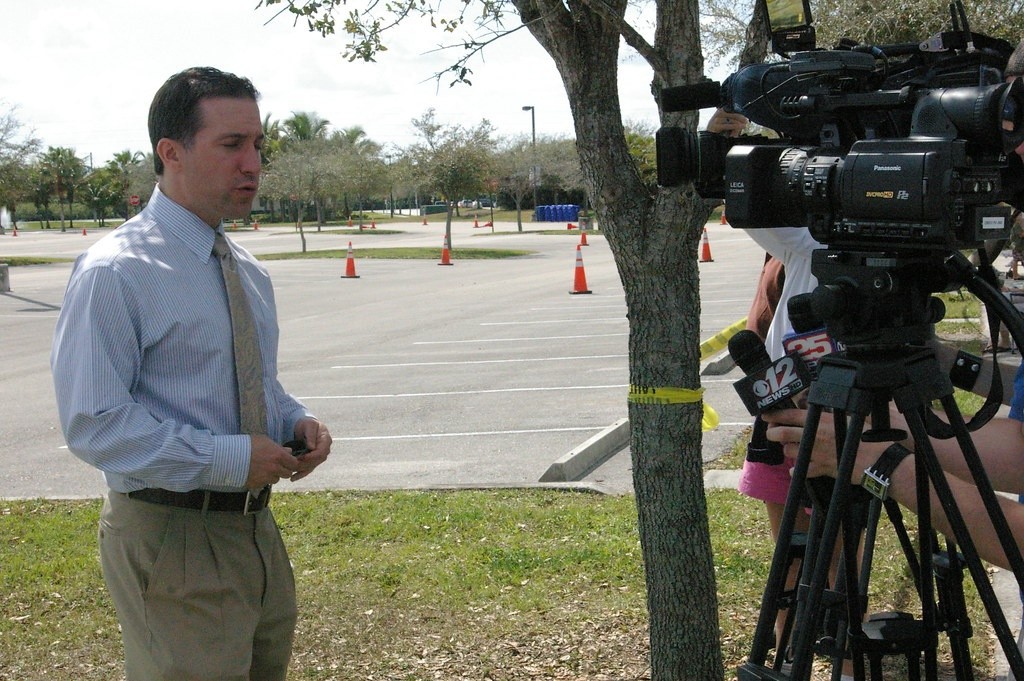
[124,488,271,512]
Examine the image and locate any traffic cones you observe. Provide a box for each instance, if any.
[579,222,592,246]
[369,217,378,229]
[437,235,457,265]
[253,219,260,231]
[12,226,18,237]
[472,214,481,227]
[362,225,368,229]
[82,228,88,236]
[340,240,361,278]
[699,227,715,262]
[568,224,579,230]
[422,213,429,225]
[720,208,731,225]
[348,215,357,228]
[567,245,592,296]
[481,221,493,227]
[232,219,237,229]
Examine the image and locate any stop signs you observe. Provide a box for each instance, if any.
[129,195,140,206]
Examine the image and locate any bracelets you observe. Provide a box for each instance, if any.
[1007,287,1010,292]
[859,443,915,499]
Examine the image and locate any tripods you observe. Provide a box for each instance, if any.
[736,250,1024,681]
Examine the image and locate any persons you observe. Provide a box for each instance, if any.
[50,67,332,681]
[981,286,1024,352]
[1010,211,1024,279]
[745,249,786,342]
[761,41,1024,681]
[706,108,865,681]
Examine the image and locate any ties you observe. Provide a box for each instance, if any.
[210,235,269,498]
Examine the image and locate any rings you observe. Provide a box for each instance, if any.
[725,119,730,124]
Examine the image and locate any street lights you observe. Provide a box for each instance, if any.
[386,154,402,221]
[522,105,537,213]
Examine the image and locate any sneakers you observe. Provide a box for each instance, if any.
[741,647,794,676]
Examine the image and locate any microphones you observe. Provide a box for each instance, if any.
[781,292,832,378]
[659,81,722,113]
[727,330,831,517]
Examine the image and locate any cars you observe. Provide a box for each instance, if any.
[435,197,492,208]
